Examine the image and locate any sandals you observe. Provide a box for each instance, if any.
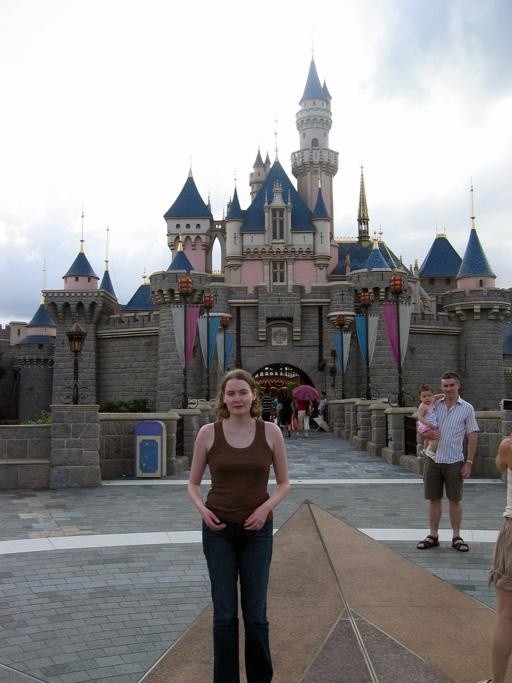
[451,536,470,553]
[417,535,440,548]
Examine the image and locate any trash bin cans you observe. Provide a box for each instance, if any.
[133,420,166,479]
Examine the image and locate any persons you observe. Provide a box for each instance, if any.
[417,383,445,459]
[488,432,512,681]
[416,369,481,550]
[255,388,327,437]
[187,367,291,683]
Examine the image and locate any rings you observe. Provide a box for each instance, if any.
[256,524,260,527]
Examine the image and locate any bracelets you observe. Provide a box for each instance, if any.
[466,459,473,464]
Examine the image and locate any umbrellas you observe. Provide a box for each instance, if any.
[292,384,320,401]
